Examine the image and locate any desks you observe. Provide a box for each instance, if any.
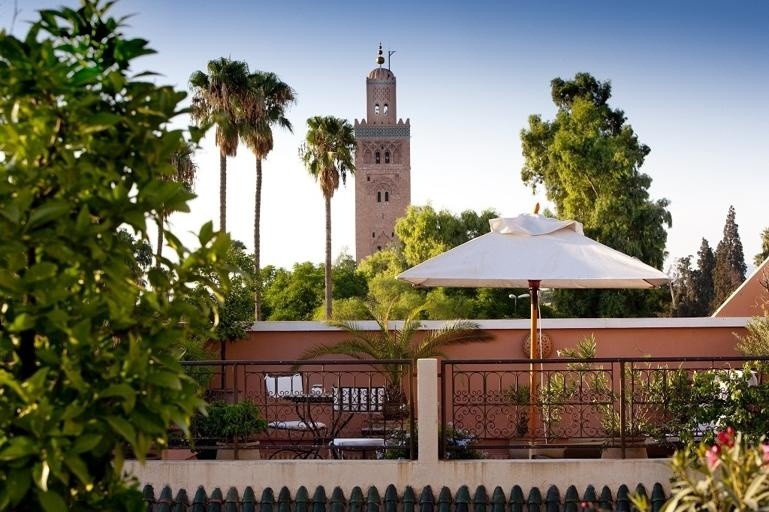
[282,394,337,458]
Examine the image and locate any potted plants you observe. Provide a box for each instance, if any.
[504,360,684,459]
[189,398,223,461]
[286,284,496,420]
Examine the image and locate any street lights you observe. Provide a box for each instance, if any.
[507,293,531,317]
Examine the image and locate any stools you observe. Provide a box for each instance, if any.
[327,438,405,459]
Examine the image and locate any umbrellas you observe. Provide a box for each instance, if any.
[394,202,679,445]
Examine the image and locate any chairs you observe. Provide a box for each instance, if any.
[262,370,323,458]
